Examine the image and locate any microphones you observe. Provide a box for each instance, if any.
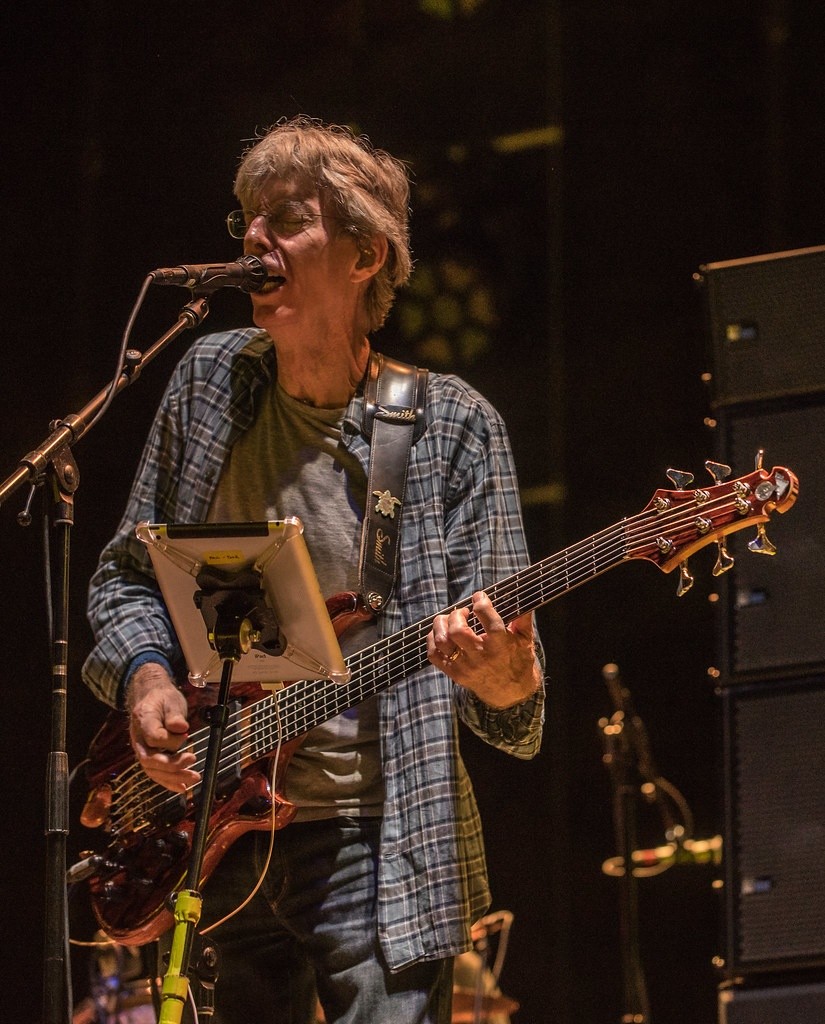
[150,255,268,293]
[471,912,512,940]
[605,837,723,869]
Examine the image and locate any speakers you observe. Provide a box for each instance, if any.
[702,246,825,1024]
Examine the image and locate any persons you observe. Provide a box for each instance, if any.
[79,113,546,1024]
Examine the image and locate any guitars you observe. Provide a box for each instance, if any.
[61,446,800,949]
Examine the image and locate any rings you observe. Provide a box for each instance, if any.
[447,647,460,662]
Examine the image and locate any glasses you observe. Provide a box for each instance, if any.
[226,210,344,239]
